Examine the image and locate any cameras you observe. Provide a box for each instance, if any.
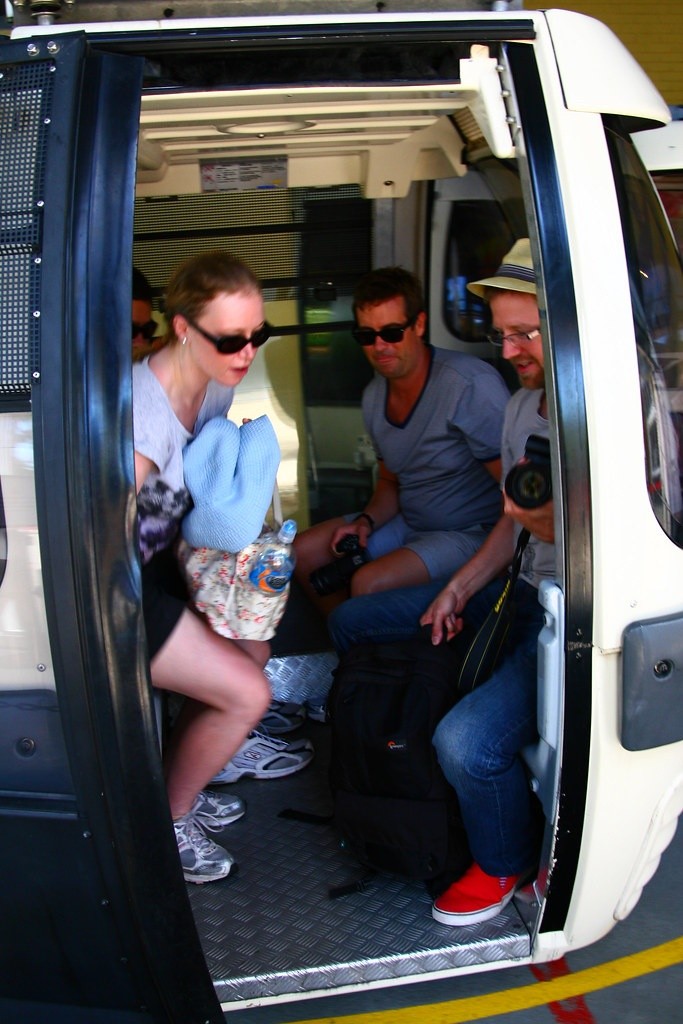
[308,535,364,597]
[505,434,554,510]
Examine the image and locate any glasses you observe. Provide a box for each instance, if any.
[351,320,413,345]
[485,327,541,349]
[131,319,158,340]
[187,321,271,354]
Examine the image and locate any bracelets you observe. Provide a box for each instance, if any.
[354,513,376,536]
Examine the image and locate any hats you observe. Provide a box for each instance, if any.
[465,238,536,299]
[181,414,281,554]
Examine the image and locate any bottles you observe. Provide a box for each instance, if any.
[249,519,298,596]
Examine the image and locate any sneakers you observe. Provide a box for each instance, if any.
[306,699,334,722]
[208,729,317,784]
[432,859,540,927]
[173,811,239,883]
[189,789,247,827]
[256,699,306,734]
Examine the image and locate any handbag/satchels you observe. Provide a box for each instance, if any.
[177,522,290,641]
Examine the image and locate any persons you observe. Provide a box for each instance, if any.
[281,268,511,721]
[131,267,315,784]
[421,238,558,926]
[131,252,272,881]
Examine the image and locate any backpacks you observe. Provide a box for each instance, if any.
[328,624,459,882]
